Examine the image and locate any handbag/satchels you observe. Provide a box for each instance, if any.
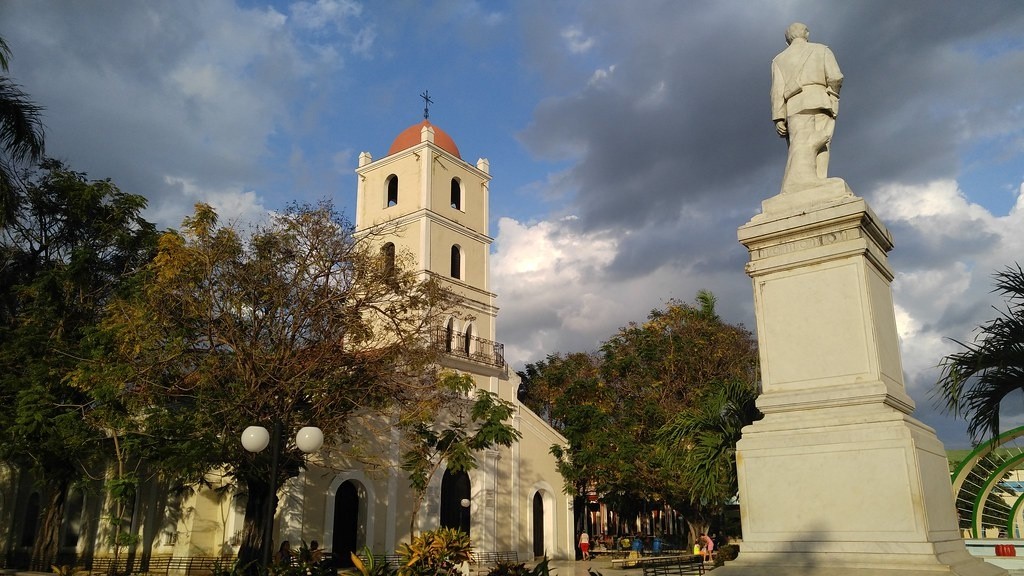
[702,547,706,553]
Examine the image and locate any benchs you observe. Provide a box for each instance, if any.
[643,555,705,576]
[612,556,669,568]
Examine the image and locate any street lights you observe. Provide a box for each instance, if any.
[241,389,324,576]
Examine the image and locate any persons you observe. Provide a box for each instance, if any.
[578,529,591,561]
[694,533,714,563]
[307,540,337,576]
[769,22,844,191]
[275,541,307,576]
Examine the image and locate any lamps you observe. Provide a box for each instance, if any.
[460,498,469,508]
